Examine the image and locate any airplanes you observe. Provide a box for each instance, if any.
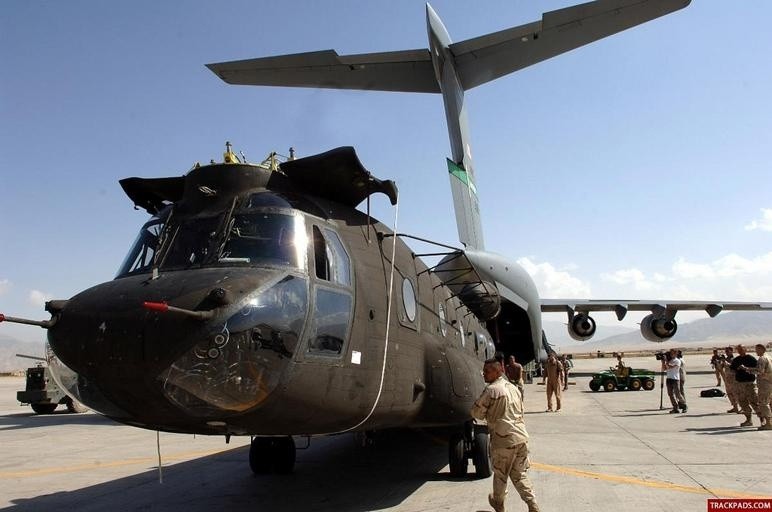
[201,0,771,369]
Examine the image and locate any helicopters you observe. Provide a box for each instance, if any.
[0,143,512,481]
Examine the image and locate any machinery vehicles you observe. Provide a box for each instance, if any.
[15,335,92,415]
[587,366,655,392]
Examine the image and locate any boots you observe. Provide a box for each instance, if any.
[670,402,688,414]
[546,407,553,412]
[489,492,505,512]
[528,504,540,512]
[555,407,560,412]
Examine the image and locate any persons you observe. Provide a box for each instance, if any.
[675,349,687,409]
[737,344,772,430]
[469,357,541,511]
[728,344,767,426]
[719,346,747,413]
[559,353,574,392]
[709,348,726,387]
[614,354,625,374]
[662,347,688,414]
[503,355,525,402]
[542,354,566,412]
